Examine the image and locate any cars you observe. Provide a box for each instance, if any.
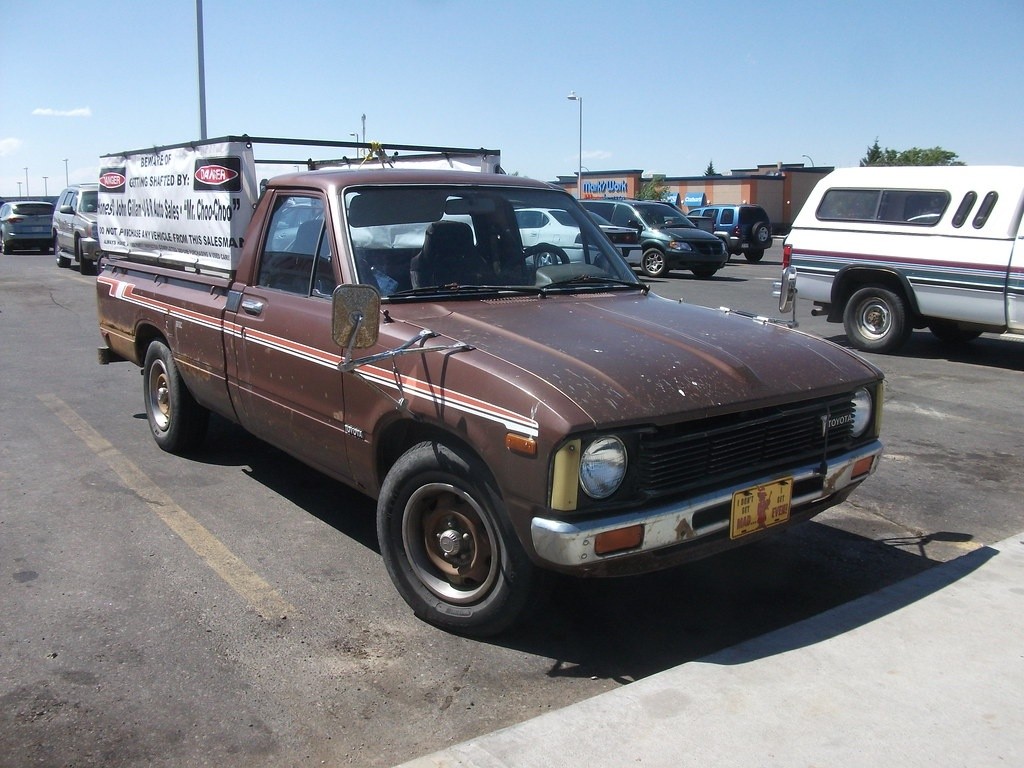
[577,198,729,278]
[513,208,643,269]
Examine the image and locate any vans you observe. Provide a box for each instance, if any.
[778,164,1024,353]
[641,199,730,247]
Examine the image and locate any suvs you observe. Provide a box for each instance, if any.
[688,204,773,264]
[0,201,55,255]
[52,181,104,275]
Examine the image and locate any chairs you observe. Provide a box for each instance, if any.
[410,220,502,291]
[289,217,384,305]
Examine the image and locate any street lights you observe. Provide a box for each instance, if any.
[42,176,48,196]
[62,158,69,187]
[361,112,366,158]
[16,182,22,197]
[23,167,29,196]
[801,154,815,168]
[350,131,360,160]
[566,90,582,198]
[294,165,300,172]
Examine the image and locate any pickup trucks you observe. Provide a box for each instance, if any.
[94,166,885,632]
